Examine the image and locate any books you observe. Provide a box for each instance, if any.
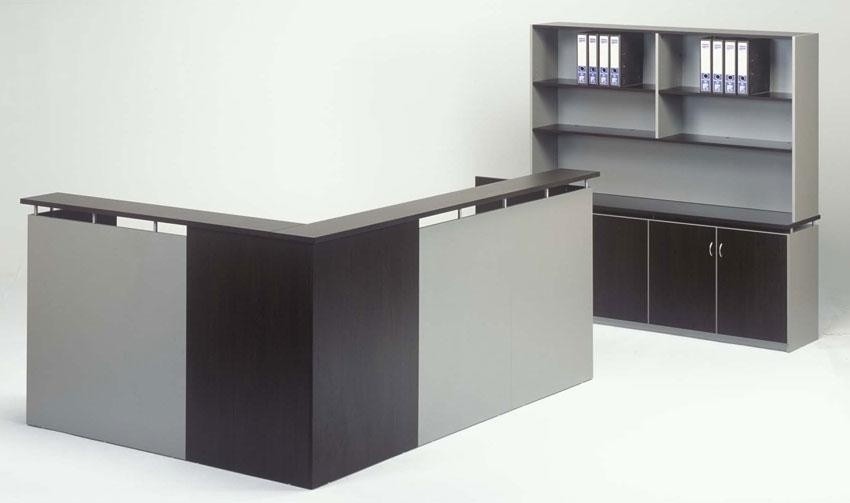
[576,31,643,86]
[700,36,772,96]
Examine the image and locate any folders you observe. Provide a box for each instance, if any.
[576,32,642,86]
[701,35,769,95]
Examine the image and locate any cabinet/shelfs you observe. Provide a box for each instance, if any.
[530,21,820,353]
